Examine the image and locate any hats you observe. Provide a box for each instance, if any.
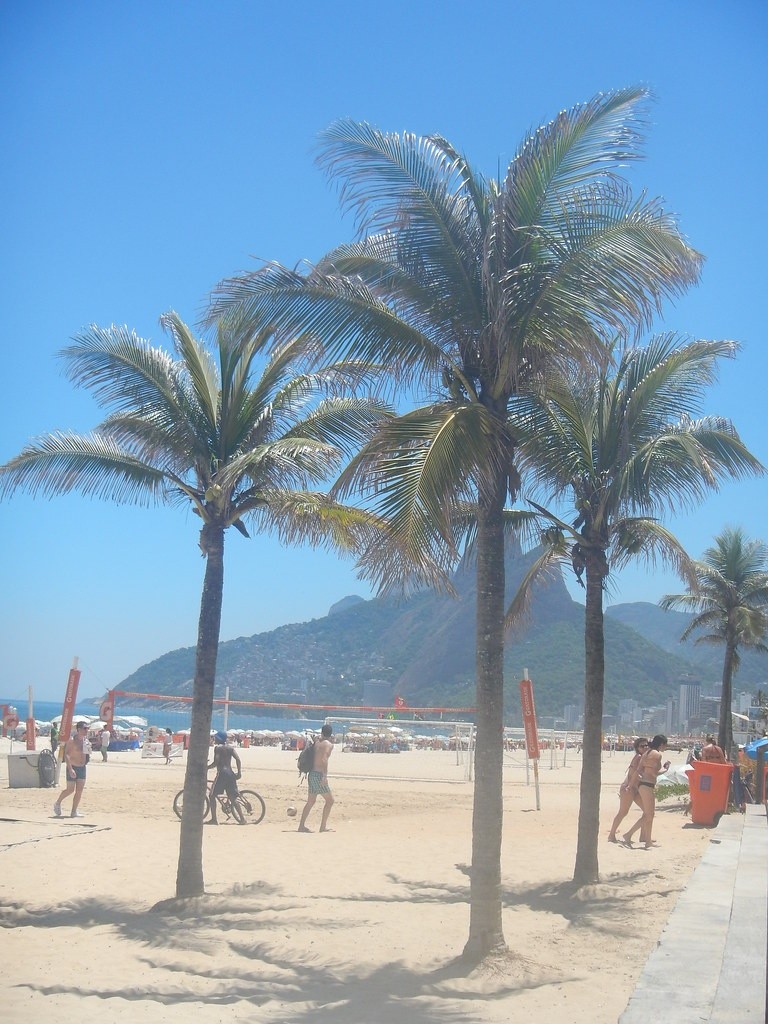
[214,730,227,742]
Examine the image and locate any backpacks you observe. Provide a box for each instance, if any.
[297,741,318,773]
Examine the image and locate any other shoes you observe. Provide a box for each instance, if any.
[54,803,61,815]
[71,812,84,818]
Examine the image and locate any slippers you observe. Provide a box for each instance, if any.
[320,828,335,832]
[298,828,315,833]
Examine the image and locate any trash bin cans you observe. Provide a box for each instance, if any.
[686,761,734,826]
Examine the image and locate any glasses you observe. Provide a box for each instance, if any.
[84,727,90,730]
[639,745,648,748]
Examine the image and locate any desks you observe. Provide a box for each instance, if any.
[107,741,140,752]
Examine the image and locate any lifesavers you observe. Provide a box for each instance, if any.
[3,713,19,731]
[98,700,112,722]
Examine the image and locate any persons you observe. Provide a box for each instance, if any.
[203,730,247,825]
[51,722,58,755]
[298,725,333,833]
[702,734,726,764]
[343,732,476,753]
[608,734,671,847]
[163,728,172,765]
[100,725,110,762]
[504,735,718,753]
[54,721,92,817]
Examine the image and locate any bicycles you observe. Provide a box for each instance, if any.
[173,759,266,825]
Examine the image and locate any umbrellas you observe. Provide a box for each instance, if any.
[0,714,148,752]
[146,726,342,751]
